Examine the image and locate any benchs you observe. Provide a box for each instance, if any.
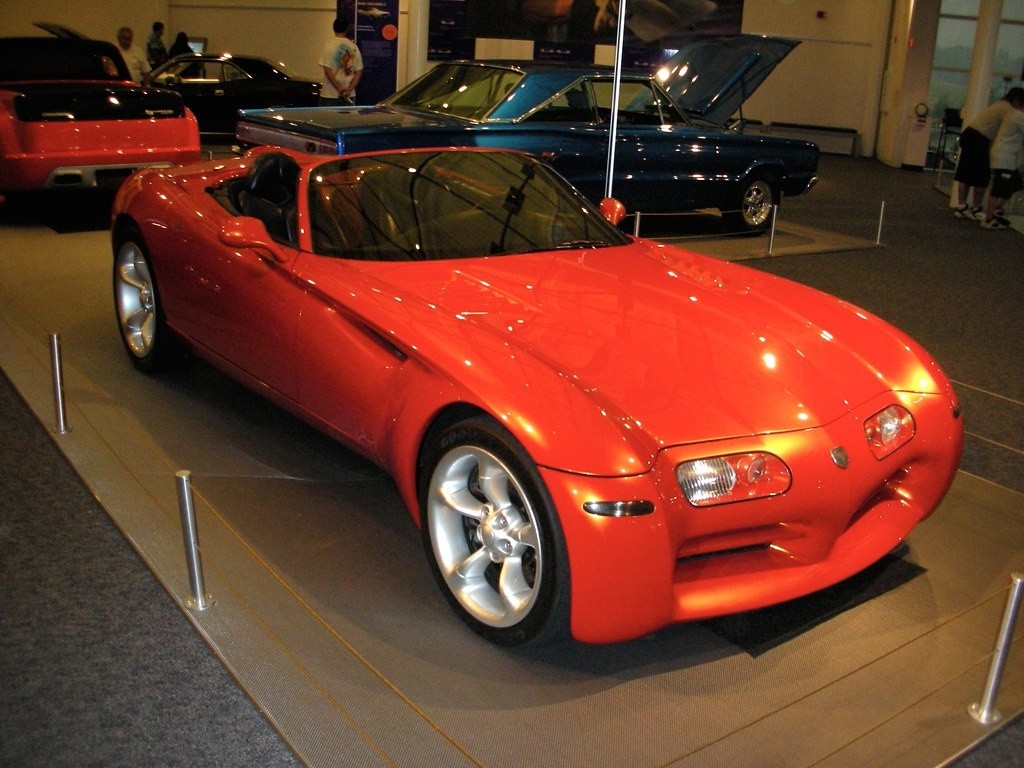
[727,119,860,158]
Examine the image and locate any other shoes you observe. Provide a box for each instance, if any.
[969,207,986,220]
[953,207,971,217]
[994,209,1010,220]
[981,213,1011,230]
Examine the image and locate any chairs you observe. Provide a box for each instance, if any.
[564,88,590,121]
[240,151,365,258]
[381,158,457,241]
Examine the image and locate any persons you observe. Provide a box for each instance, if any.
[318,18,364,107]
[168,32,195,74]
[146,22,167,69]
[954,87,1024,220]
[107,27,149,87]
[981,90,1024,228]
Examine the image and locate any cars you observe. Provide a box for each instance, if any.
[234,31,822,239]
[0,22,324,212]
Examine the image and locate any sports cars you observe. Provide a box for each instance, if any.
[106,141,966,654]
